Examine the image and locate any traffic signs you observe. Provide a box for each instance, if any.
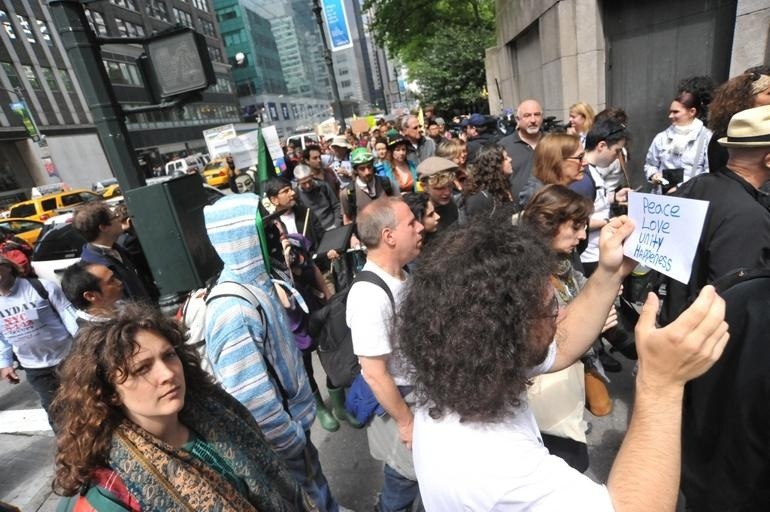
[0,85,66,185]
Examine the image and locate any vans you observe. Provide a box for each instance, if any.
[287,133,324,156]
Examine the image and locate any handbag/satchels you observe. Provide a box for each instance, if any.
[585,367,612,416]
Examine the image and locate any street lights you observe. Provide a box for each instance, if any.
[234,48,264,127]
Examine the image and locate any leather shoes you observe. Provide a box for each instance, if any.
[599,353,621,372]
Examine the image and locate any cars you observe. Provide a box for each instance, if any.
[2,152,242,292]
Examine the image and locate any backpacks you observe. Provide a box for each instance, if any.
[0,242,30,276]
[308,270,395,386]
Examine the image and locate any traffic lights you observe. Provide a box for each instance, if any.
[142,24,219,100]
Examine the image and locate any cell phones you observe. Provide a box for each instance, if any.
[635,186,643,192]
[7,374,19,383]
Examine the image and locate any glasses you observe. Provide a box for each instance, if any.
[567,154,582,162]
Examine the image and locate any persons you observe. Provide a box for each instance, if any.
[0,63,769,510]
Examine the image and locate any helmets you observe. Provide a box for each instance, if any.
[350,147,374,167]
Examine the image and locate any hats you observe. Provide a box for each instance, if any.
[330,136,351,149]
[717,105,770,148]
[416,156,458,181]
[293,164,313,182]
[388,134,407,148]
[462,113,487,125]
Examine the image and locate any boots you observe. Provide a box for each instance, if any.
[312,386,364,431]
